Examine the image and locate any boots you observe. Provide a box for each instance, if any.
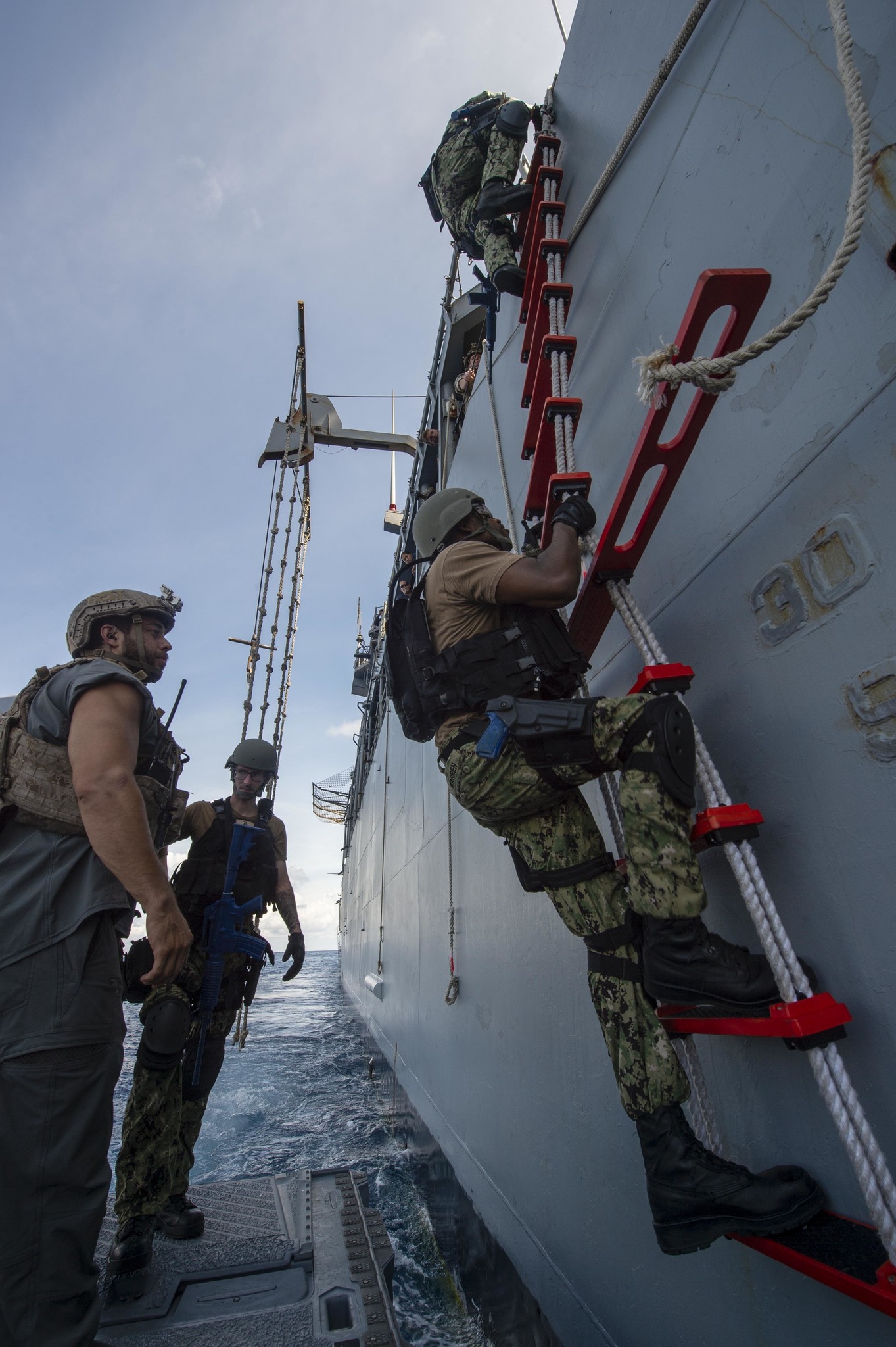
[475,178,536,221]
[642,915,816,1010]
[637,1107,825,1256]
[492,264,527,298]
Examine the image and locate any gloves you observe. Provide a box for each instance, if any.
[282,932,305,982]
[520,521,545,552]
[550,494,597,535]
[531,105,542,133]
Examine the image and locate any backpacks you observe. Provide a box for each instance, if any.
[385,557,440,742]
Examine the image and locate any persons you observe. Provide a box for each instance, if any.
[401,550,416,577]
[105,738,307,1306]
[383,487,830,1256]
[396,579,413,601]
[414,487,437,502]
[417,90,539,299]
[374,609,384,626]
[0,584,195,1347]
[454,342,482,414]
[422,429,439,446]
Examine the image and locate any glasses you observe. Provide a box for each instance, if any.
[475,504,493,518]
[233,766,269,782]
[400,583,409,589]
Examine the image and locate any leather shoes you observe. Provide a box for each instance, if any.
[154,1195,205,1238]
[105,1214,155,1276]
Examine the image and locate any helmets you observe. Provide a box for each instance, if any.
[224,738,278,776]
[466,348,482,361]
[65,590,175,659]
[412,487,485,567]
